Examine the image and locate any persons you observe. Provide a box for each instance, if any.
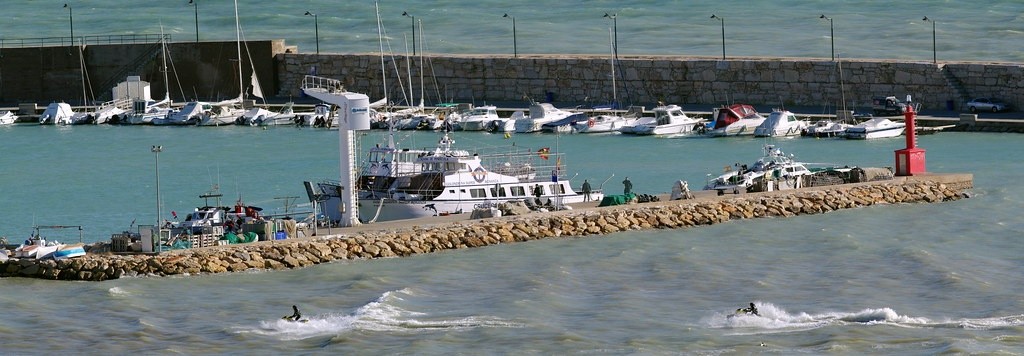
[288,305,301,320]
[745,302,758,316]
[582,180,591,202]
[534,184,541,196]
[623,178,632,194]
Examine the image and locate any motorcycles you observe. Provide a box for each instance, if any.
[281,315,310,326]
[728,308,761,320]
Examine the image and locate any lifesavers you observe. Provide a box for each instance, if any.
[206,111,211,117]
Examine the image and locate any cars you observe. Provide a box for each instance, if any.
[966,97,1007,113]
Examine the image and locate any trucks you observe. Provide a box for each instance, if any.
[872,96,907,115]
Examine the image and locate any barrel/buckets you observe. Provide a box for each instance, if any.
[276,230,285,240]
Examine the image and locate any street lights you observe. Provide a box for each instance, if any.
[402,11,416,55]
[502,11,517,57]
[709,13,726,60]
[819,14,835,60]
[187,0,199,43]
[304,10,320,53]
[603,12,618,59]
[63,3,74,45]
[922,15,937,63]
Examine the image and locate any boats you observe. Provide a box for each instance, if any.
[79,102,907,140]
[705,145,855,192]
[168,205,261,234]
[17,239,88,260]
[303,144,605,225]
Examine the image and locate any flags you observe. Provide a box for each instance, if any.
[537,147,550,160]
[557,157,560,170]
